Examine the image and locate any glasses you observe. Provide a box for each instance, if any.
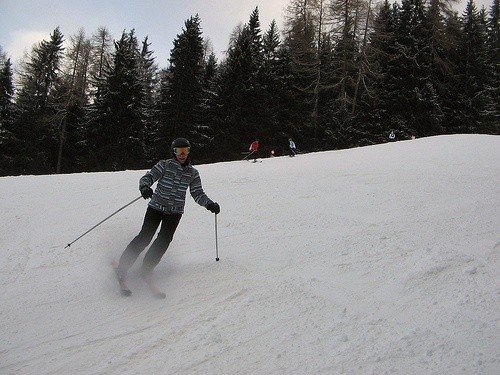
[173,147,190,154]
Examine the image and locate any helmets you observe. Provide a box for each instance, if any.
[171,138,192,161]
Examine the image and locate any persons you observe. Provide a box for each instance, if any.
[248,139,259,163]
[288,138,296,157]
[117,137,220,283]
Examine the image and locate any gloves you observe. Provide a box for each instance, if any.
[140,185,153,200]
[206,201,220,215]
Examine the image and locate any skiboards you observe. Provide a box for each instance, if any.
[284,155,298,158]
[246,159,263,163]
[110,261,166,299]
